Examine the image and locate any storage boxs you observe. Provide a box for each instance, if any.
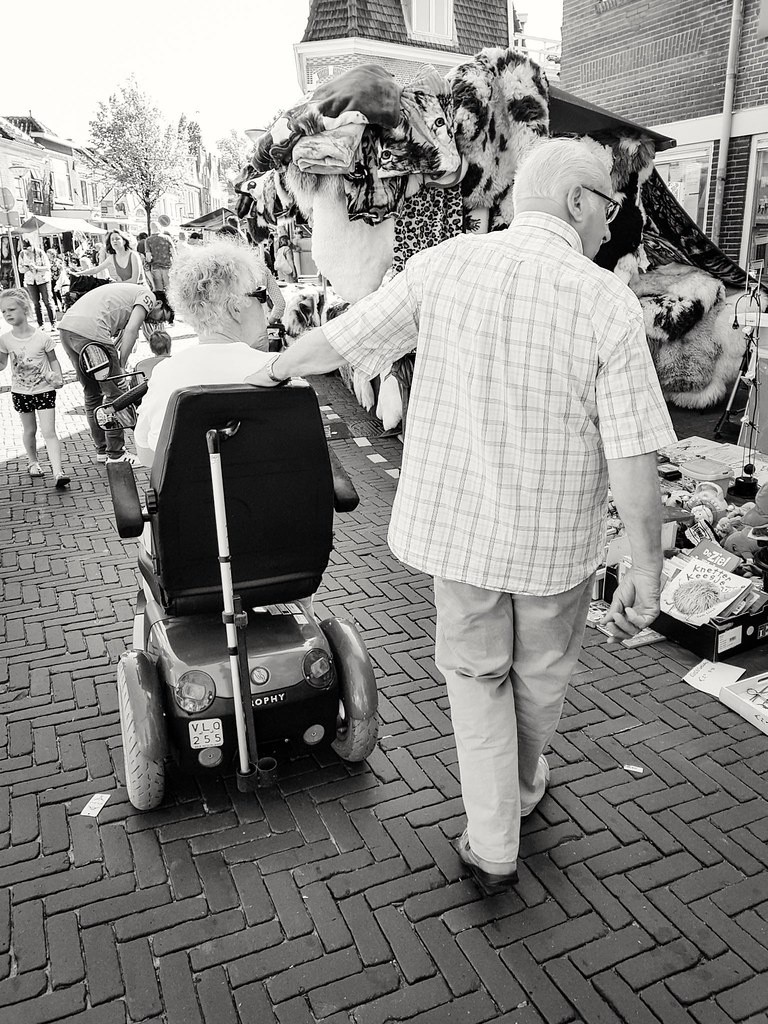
[679,458,734,498]
[603,563,768,663]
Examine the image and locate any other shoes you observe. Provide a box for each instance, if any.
[56,474,71,488]
[458,828,520,887]
[519,754,550,817]
[26,462,45,476]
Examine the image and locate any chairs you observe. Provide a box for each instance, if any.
[105,380,357,615]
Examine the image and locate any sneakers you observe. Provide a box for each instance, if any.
[96,452,145,469]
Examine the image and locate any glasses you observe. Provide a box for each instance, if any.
[566,183,619,224]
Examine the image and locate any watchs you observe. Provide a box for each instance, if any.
[266,352,290,383]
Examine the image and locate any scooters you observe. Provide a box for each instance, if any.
[61,268,114,311]
[76,341,379,811]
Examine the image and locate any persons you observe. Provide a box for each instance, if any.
[135,232,156,291]
[274,235,298,284]
[69,228,149,354]
[241,135,678,894]
[145,221,177,291]
[0,288,71,487]
[57,283,175,468]
[47,249,68,313]
[134,234,286,546]
[18,238,58,332]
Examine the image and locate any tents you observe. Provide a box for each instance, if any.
[11,215,109,249]
